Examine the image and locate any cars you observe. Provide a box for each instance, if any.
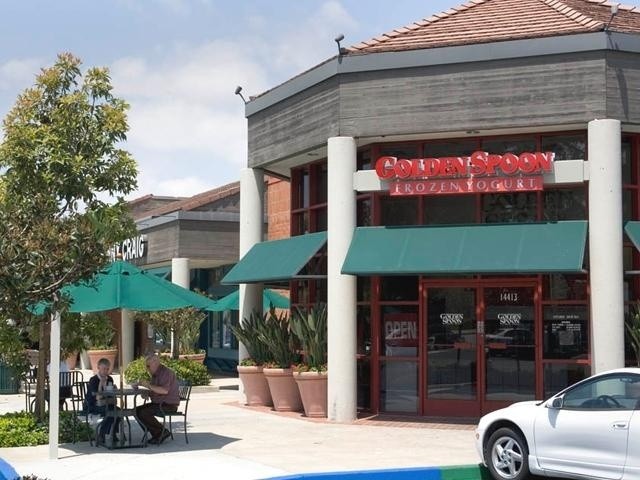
[485,321,585,343]
[474,367,640,480]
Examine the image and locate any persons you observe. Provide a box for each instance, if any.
[85,358,123,445]
[132,356,179,446]
[43,360,73,411]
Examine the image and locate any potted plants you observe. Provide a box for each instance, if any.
[60,312,118,374]
[225,299,327,417]
[153,287,213,365]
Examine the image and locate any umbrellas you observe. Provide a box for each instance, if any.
[205,286,291,313]
[25,251,223,445]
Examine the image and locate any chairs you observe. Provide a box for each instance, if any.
[28,371,192,447]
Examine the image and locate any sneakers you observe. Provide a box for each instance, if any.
[148,427,170,445]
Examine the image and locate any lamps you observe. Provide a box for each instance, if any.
[335,34,345,55]
[605,4,618,31]
[235,86,246,103]
